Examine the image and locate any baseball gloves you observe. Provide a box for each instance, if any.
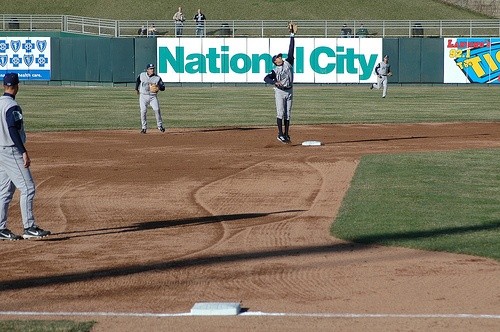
[287,23,298,34]
[149,83,159,93]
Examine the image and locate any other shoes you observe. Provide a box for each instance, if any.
[369,83,377,89]
[383,96,385,98]
[140,129,146,133]
[158,125,165,132]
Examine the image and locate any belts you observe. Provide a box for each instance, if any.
[382,75,387,76]
[5,145,17,147]
[275,84,293,91]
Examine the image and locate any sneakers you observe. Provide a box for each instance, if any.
[23,225,51,240]
[283,133,290,141]
[0,228,22,240]
[277,135,288,144]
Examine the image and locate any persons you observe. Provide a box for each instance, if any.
[342,23,352,37]
[264,25,294,145]
[137,23,158,38]
[135,62,166,134]
[370,54,392,98]
[173,6,186,37]
[0,73,51,242]
[358,23,369,38]
[194,8,207,38]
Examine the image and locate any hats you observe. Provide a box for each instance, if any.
[4,72,20,86]
[147,63,154,68]
[272,54,282,62]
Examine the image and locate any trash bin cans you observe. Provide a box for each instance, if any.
[8,17,19,30]
[221,24,230,38]
[413,23,424,38]
